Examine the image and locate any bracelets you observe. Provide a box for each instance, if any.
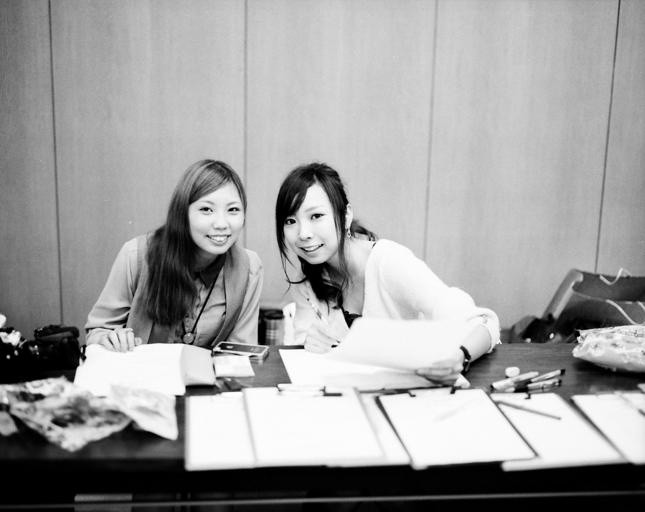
[459,344,472,362]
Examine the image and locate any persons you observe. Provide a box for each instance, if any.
[272,161,502,386]
[82,158,266,352]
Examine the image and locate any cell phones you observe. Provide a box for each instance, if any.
[213,341,270,362]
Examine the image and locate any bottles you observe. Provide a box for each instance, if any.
[262,308,285,345]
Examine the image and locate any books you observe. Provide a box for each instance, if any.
[85,342,217,386]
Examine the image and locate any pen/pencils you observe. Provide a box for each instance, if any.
[490,369,566,393]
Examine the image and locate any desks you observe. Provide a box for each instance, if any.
[0,342,644,511]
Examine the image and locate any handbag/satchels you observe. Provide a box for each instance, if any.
[541,268,644,337]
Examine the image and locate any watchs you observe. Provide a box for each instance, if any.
[460,346,472,374]
